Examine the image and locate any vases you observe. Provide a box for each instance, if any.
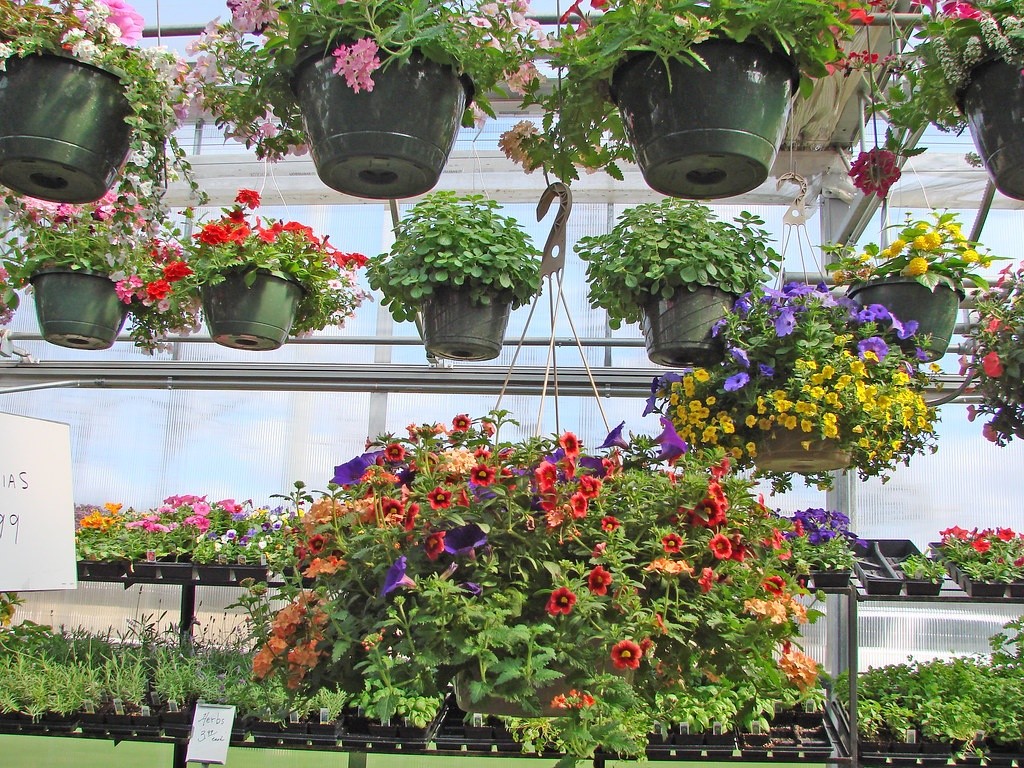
[746,422,851,472]
[27,267,129,351]
[954,46,1024,202]
[288,39,476,199]
[846,276,966,363]
[607,27,800,199]
[0,50,139,203]
[202,268,310,351]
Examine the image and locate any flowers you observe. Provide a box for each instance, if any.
[0,2,1024,768]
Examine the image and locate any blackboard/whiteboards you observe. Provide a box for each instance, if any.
[0,412,78,592]
[184,703,236,765]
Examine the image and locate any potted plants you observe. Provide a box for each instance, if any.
[368,193,544,361]
[574,195,785,367]
[0,619,345,730]
[833,615,1024,767]
